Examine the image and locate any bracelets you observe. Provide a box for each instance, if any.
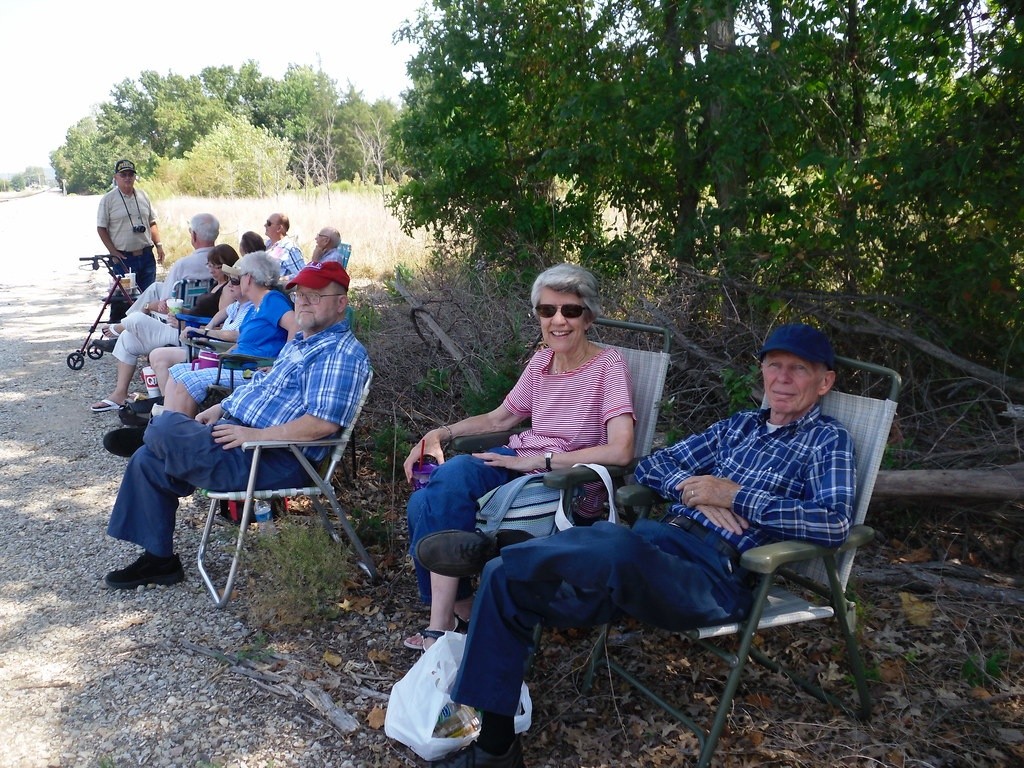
[156,243,162,247]
[439,426,452,443]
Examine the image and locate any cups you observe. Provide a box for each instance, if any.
[120,273,137,296]
[191,350,222,371]
[412,462,440,492]
[142,366,162,398]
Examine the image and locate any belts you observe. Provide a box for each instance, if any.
[666,515,740,563]
[117,246,152,257]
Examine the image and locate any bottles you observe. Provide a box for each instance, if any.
[166,291,183,315]
[253,499,279,539]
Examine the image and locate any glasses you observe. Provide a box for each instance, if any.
[535,304,589,318]
[189,228,196,242]
[267,220,279,227]
[288,291,342,305]
[118,172,134,178]
[228,276,240,285]
[205,263,222,271]
[317,233,327,238]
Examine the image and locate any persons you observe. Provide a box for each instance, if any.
[403,265,638,658]
[90,214,345,423]
[416,327,857,768]
[104,261,369,589]
[96,159,166,322]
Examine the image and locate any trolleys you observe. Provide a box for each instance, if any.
[66,255,144,371]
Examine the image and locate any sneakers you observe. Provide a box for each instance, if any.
[105,553,185,589]
[104,428,146,457]
[416,527,536,578]
[431,736,523,768]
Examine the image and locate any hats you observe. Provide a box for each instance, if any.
[285,261,350,292]
[222,259,241,277]
[114,159,137,174]
[755,324,836,371]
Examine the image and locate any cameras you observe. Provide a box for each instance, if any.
[133,225,146,233]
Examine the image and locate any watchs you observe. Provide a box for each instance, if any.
[545,452,553,472]
[144,302,151,312]
[204,328,209,336]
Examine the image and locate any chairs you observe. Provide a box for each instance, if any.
[202,369,383,608]
[150,241,349,409]
[582,355,901,767]
[447,313,671,675]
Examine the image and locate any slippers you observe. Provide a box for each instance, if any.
[404,617,460,656]
[91,399,128,411]
[102,324,121,338]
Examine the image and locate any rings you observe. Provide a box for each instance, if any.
[692,489,695,497]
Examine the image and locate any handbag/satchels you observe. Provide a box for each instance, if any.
[220,495,289,526]
[384,630,532,760]
